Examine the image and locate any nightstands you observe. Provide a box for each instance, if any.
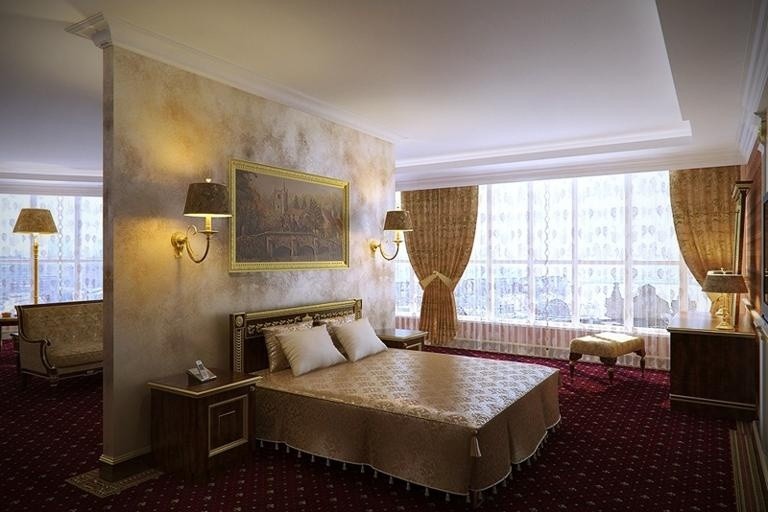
[373,327,428,354]
[147,367,263,486]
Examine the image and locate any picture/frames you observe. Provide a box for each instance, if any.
[228,156,351,275]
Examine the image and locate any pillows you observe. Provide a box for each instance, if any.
[317,313,356,355]
[276,324,348,377]
[331,317,388,363]
[261,320,314,373]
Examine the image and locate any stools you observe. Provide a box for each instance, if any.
[570,332,646,388]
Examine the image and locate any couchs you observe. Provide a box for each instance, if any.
[11,300,104,395]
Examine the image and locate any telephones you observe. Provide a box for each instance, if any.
[187,358,217,382]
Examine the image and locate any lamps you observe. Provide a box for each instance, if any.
[702,273,749,330]
[171,182,232,263]
[370,210,414,261]
[12,209,58,304]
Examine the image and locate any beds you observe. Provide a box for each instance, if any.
[228,297,564,510]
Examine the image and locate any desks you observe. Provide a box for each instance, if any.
[666,327,759,422]
[0,315,18,352]
